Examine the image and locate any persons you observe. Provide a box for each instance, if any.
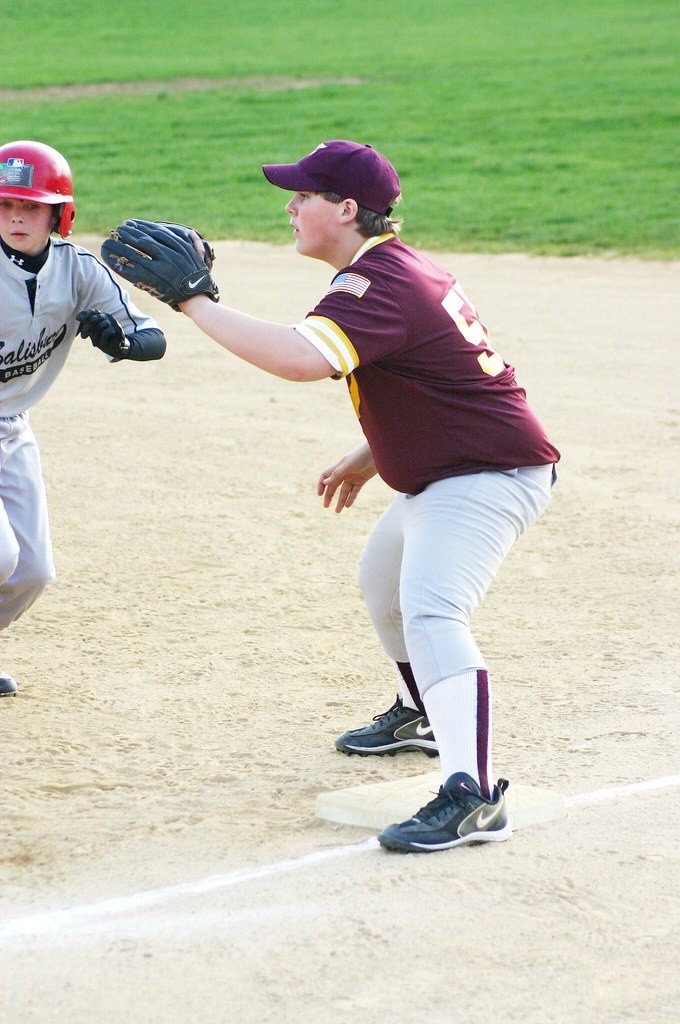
[100,141,561,851]
[0,139,166,701]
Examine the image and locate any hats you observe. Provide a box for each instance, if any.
[262,140,401,219]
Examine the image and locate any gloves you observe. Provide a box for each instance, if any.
[75,308,125,357]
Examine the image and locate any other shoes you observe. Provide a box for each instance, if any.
[0,671,18,696]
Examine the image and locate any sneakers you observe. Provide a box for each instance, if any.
[335,694,439,758]
[377,772,512,854]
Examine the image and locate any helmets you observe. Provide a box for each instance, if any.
[0,141,76,240]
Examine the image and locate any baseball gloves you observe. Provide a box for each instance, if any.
[100,217,220,314]
